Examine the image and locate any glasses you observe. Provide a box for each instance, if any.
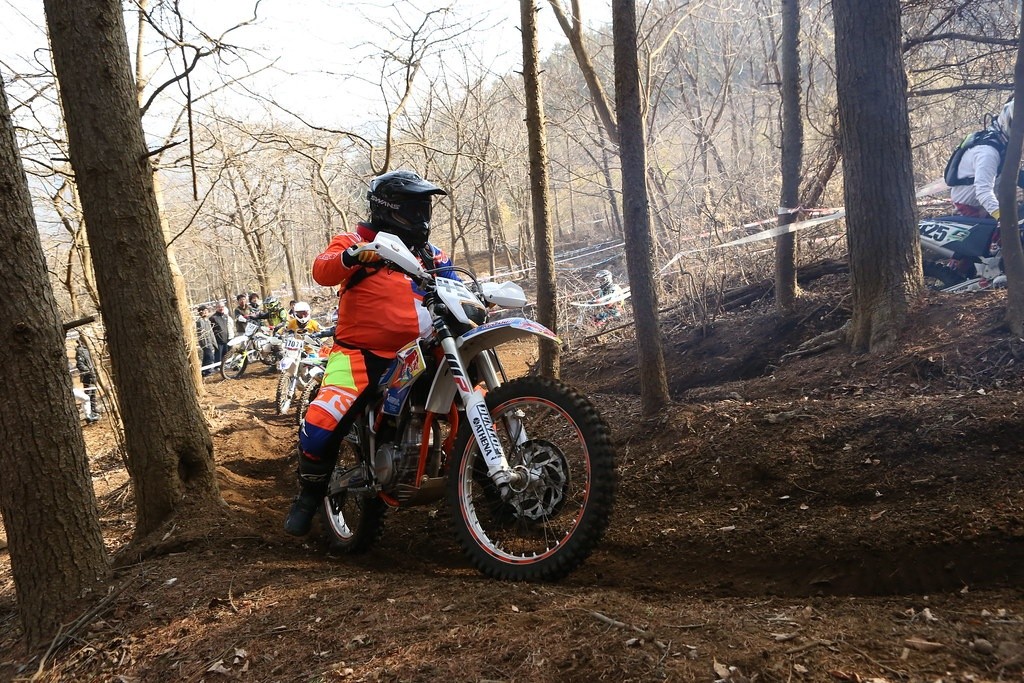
[391,199,433,224]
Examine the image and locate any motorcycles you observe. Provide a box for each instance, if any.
[314,231,618,585]
[274,331,333,427]
[221,312,282,380]
[917,202,1024,295]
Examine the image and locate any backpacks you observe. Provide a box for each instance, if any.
[943,128,1007,187]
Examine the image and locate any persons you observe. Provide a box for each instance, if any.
[73,334,103,424]
[951,98,1023,249]
[283,168,463,540]
[196,288,340,391]
[590,270,625,334]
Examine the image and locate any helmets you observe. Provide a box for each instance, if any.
[366,170,448,250]
[594,270,613,288]
[264,296,282,312]
[997,97,1015,137]
[294,302,311,325]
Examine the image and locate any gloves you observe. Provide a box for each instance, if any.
[342,242,380,268]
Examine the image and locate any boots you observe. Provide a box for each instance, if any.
[284,444,338,537]
